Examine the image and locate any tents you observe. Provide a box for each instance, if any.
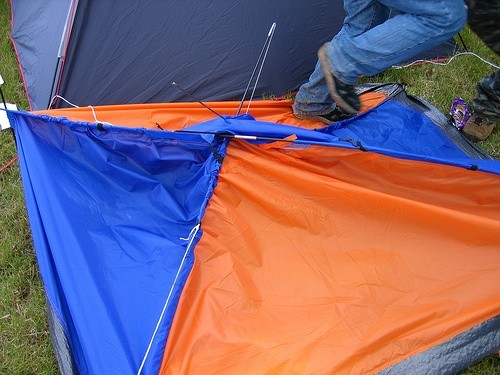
[0,83,500,375]
[8,0,347,110]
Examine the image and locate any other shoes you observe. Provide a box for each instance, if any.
[318,42,362,115]
[292,104,351,126]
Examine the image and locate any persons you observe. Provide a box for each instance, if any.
[292,0,468,124]
[460,0,500,140]
